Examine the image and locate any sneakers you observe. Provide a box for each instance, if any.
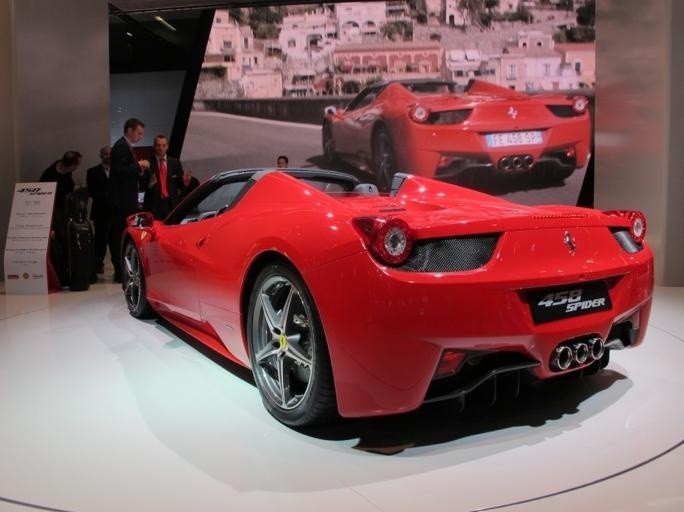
[60,265,121,289]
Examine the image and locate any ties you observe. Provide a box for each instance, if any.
[160,157,170,200]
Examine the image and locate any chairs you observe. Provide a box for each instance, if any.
[349,182,380,197]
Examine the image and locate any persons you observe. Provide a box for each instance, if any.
[277,155,289,168]
[107,119,150,285]
[86,143,113,273]
[37,148,82,289]
[140,133,192,221]
[181,174,204,212]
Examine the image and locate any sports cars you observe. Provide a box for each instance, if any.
[322,80,592,188]
[119,167,655,428]
